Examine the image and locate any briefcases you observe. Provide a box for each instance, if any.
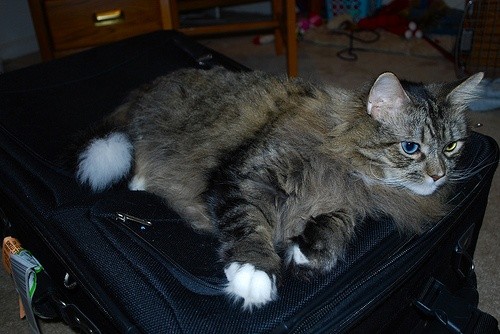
[0,30,499,332]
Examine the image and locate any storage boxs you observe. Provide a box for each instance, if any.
[2,27,500,334]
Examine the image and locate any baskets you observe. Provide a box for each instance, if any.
[328,0,381,22]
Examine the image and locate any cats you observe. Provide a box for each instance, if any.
[75,63,485,312]
